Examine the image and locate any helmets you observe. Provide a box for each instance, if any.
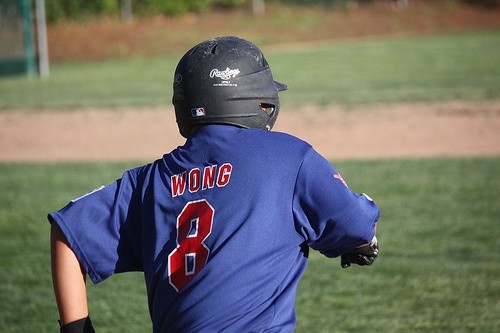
[173,37,288,140]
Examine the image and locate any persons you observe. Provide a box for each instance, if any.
[47,35,379,333]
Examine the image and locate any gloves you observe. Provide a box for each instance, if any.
[341,241,380,268]
[58,320,95,333]
